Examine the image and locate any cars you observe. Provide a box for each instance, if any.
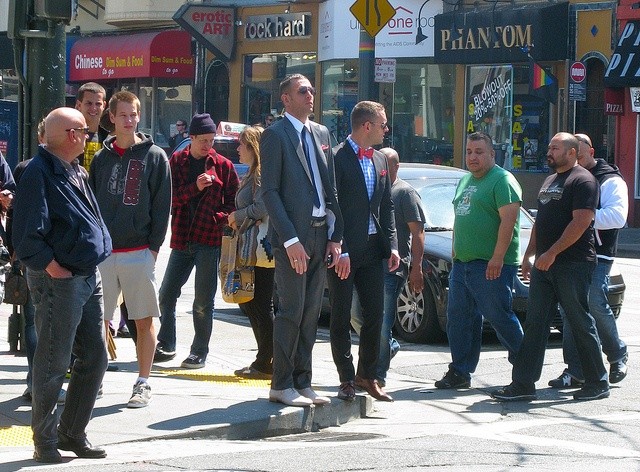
[167,134,262,179]
[237,164,625,343]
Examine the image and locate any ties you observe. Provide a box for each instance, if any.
[301,125,320,209]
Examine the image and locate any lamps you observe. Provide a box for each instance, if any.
[415,0,429,45]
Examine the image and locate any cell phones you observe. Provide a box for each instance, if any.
[327,254,332,269]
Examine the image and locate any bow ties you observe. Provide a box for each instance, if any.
[358,148,373,159]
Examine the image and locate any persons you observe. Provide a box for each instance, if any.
[89,91,172,408]
[150,114,240,369]
[13,106,110,463]
[494,133,612,401]
[329,101,401,402]
[548,132,628,388]
[17,267,67,403]
[259,74,343,406]
[265,113,274,125]
[7,119,46,261]
[73,83,109,173]
[0,150,16,263]
[435,132,525,390]
[228,127,275,379]
[350,148,425,387]
[174,117,188,145]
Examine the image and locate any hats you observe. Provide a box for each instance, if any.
[188,113,216,134]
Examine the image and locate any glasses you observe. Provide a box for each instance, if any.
[65,126,89,133]
[362,122,389,129]
[298,85,317,96]
[573,135,592,148]
[176,123,186,126]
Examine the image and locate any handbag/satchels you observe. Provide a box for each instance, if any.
[239,215,275,268]
[221,224,255,303]
[0,260,28,305]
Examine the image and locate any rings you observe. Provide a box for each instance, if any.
[292,257,299,265]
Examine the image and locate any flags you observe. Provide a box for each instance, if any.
[526,57,558,105]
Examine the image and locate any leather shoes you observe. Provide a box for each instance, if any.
[338,380,356,401]
[354,374,393,401]
[56,434,107,458]
[33,445,63,463]
[269,388,313,405]
[295,388,331,403]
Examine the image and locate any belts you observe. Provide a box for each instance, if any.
[367,234,379,242]
[310,220,327,227]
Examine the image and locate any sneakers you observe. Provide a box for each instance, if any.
[492,380,537,401]
[234,367,252,375]
[107,364,118,371]
[435,371,471,390]
[117,325,132,337]
[609,353,628,383]
[153,346,176,363]
[549,373,585,388]
[23,388,32,400]
[354,377,385,393]
[96,383,103,399]
[181,354,205,368]
[573,380,610,400]
[57,389,67,404]
[126,380,153,407]
[241,367,273,380]
